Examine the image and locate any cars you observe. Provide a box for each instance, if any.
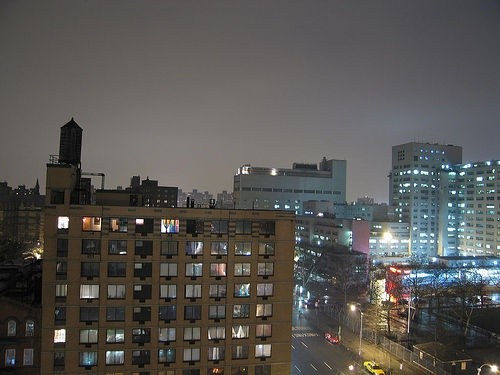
[364,360,385,375]
[304,296,328,307]
[324,332,339,344]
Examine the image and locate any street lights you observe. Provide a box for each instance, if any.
[350,305,362,357]
[477,364,498,375]
